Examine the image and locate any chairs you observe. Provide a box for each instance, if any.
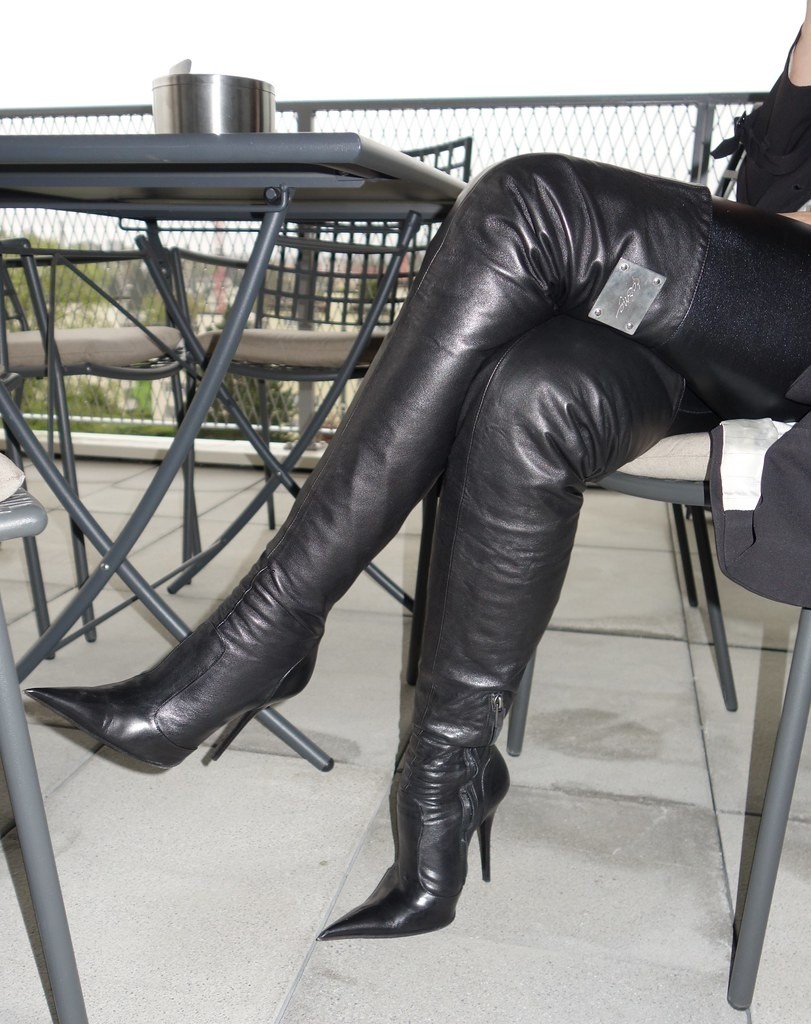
[2,136,810,1024]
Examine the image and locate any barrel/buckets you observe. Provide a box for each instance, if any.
[152,75,275,134]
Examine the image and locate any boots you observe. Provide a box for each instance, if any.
[24,152,714,942]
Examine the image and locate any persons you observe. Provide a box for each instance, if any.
[15,3,811,945]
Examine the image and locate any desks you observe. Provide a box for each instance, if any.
[1,133,471,770]
